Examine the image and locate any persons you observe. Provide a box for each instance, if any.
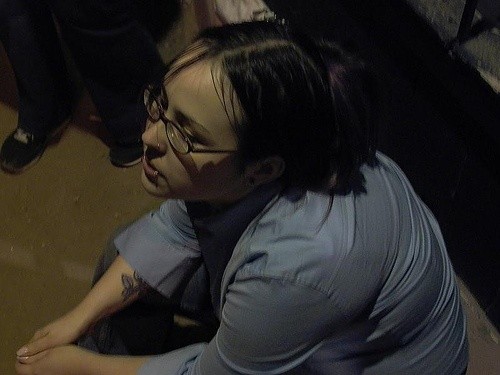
[15,17,470,375]
[0,0,174,174]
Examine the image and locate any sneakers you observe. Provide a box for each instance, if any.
[1,108,73,174]
[109,128,145,167]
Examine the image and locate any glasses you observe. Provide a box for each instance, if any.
[143,83,240,155]
